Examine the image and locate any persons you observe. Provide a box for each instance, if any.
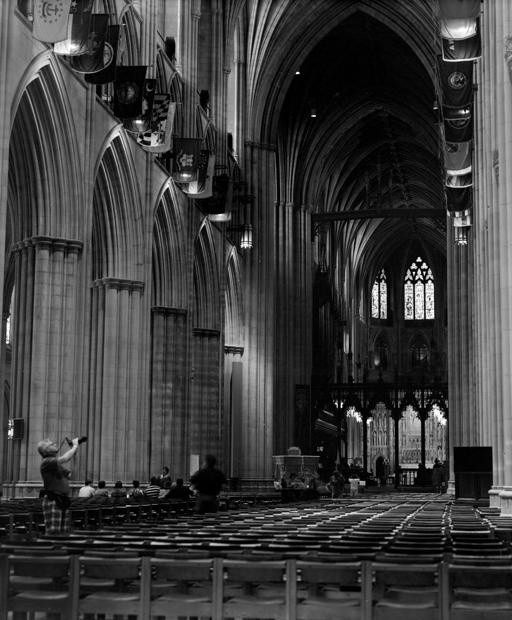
[78,479,95,500]
[110,481,127,497]
[158,465,172,482]
[95,480,110,497]
[128,480,144,498]
[159,476,193,501]
[143,474,161,503]
[347,472,361,497]
[36,434,77,537]
[190,452,228,514]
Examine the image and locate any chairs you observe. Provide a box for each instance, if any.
[1,487,512,620]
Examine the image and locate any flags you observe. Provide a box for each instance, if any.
[430,1,484,237]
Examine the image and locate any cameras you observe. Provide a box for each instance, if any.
[66,436,88,446]
